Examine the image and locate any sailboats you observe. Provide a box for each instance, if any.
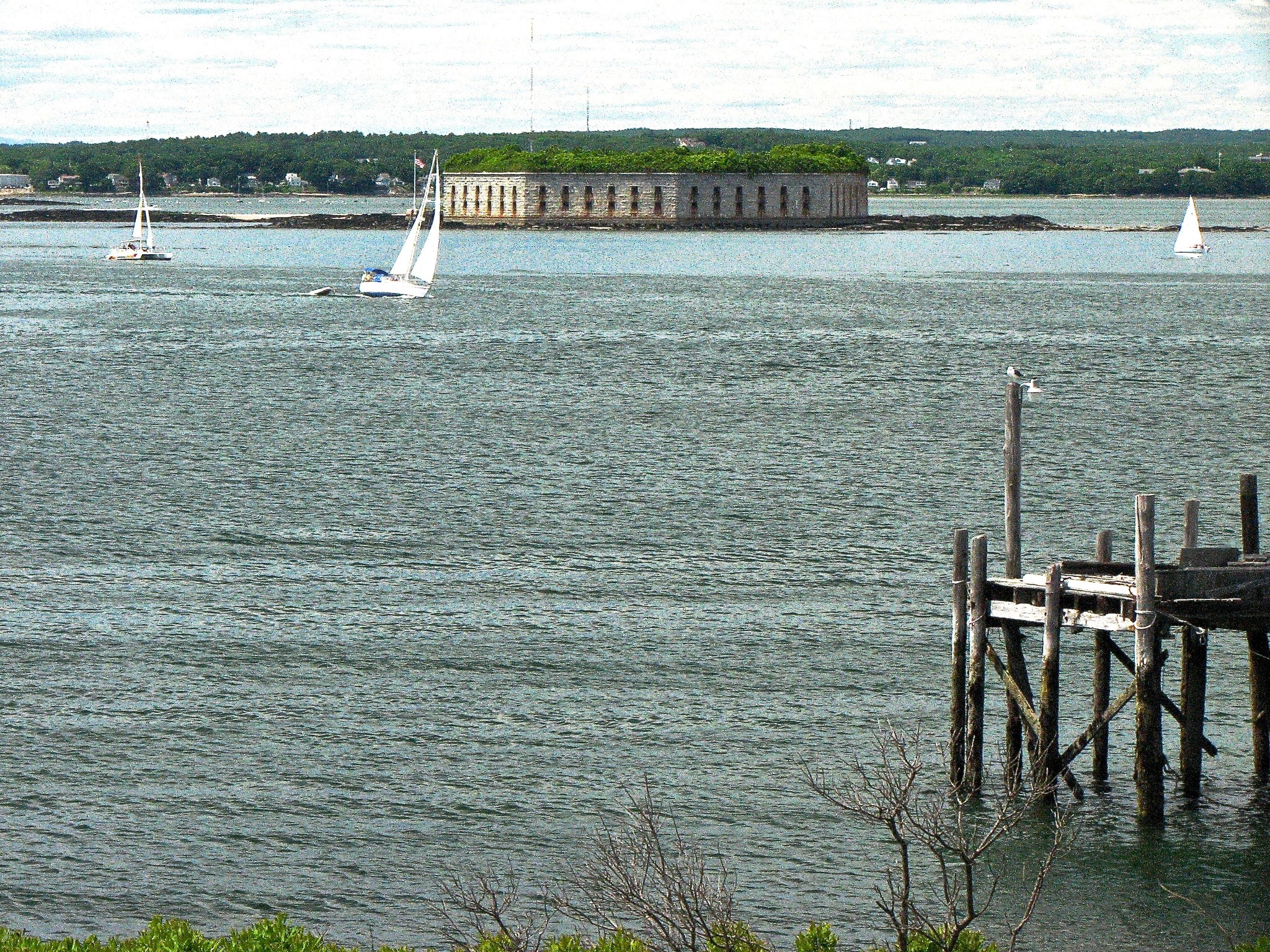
[1172,195,1210,254]
[359,149,442,298]
[106,162,173,261]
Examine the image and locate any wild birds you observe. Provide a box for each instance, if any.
[1019,379,1044,394]
[1007,366,1023,377]
